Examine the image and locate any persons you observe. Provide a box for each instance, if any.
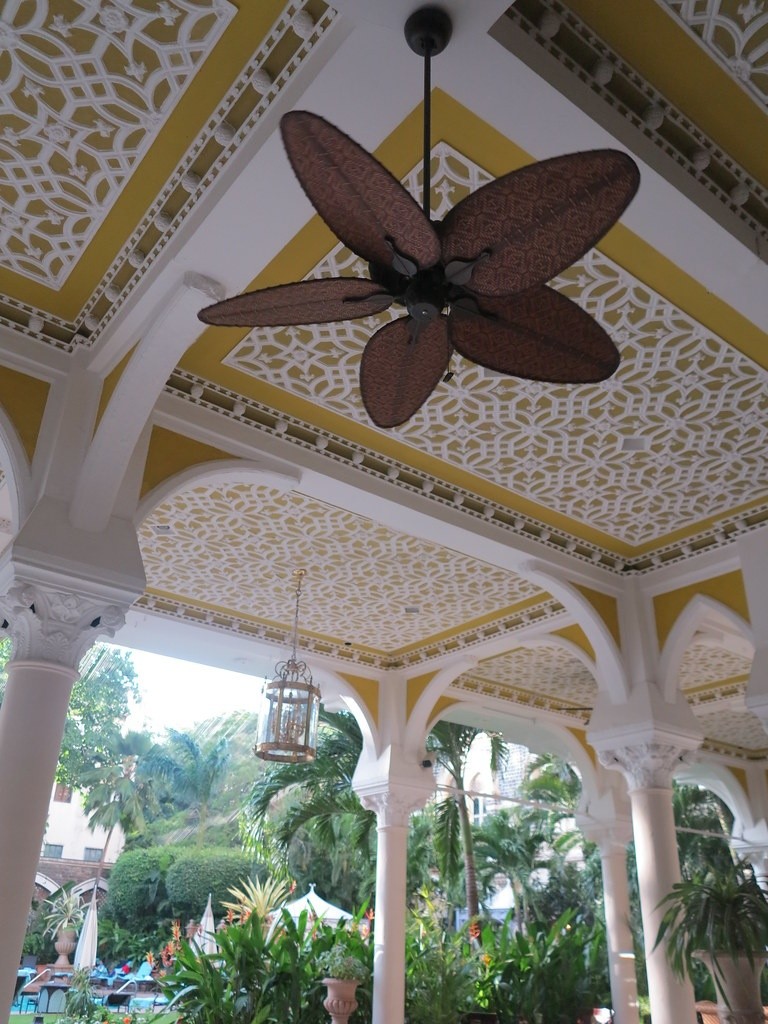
[112,960,133,979]
[149,958,159,978]
[90,959,105,978]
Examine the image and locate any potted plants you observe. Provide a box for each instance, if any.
[645,849,768,1024]
[319,956,364,1024]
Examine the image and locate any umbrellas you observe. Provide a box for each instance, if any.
[462,876,515,910]
[265,881,358,920]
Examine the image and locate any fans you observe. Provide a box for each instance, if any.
[192,6,639,433]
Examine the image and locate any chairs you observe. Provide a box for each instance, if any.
[14,959,180,1012]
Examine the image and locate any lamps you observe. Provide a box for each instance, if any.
[253,570,323,765]
